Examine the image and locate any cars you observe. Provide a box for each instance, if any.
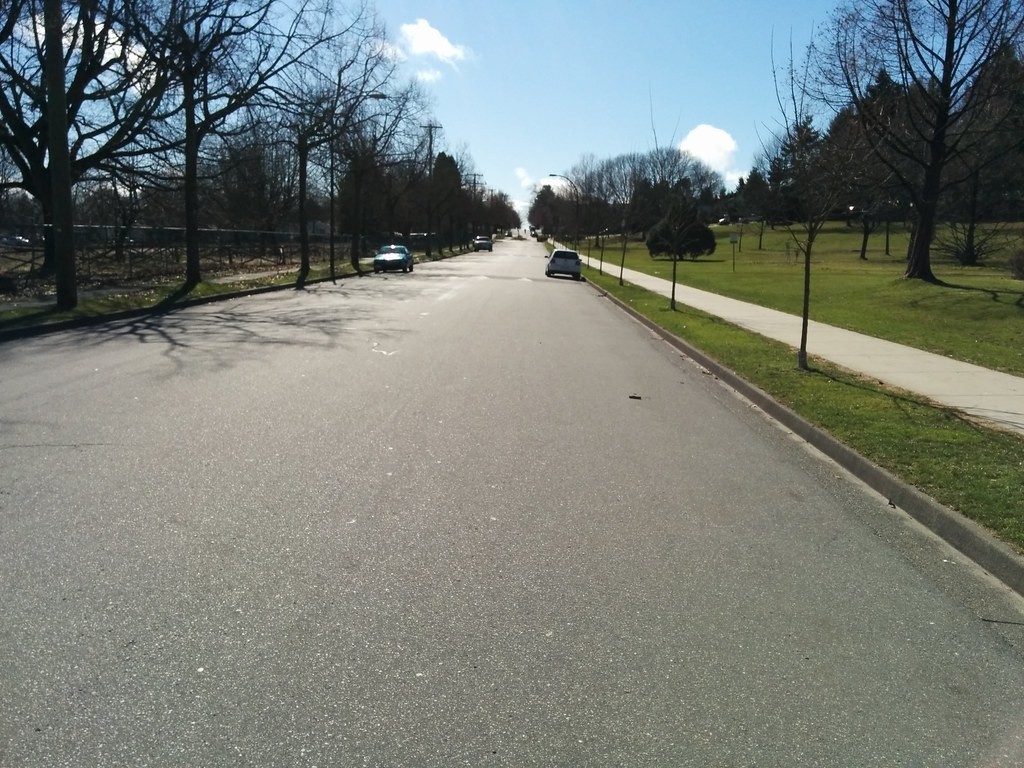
[373,245,414,273]
[537,234,545,241]
[545,249,582,279]
[473,235,492,251]
[531,231,537,236]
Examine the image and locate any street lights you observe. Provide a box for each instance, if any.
[549,174,578,250]
[331,93,387,279]
